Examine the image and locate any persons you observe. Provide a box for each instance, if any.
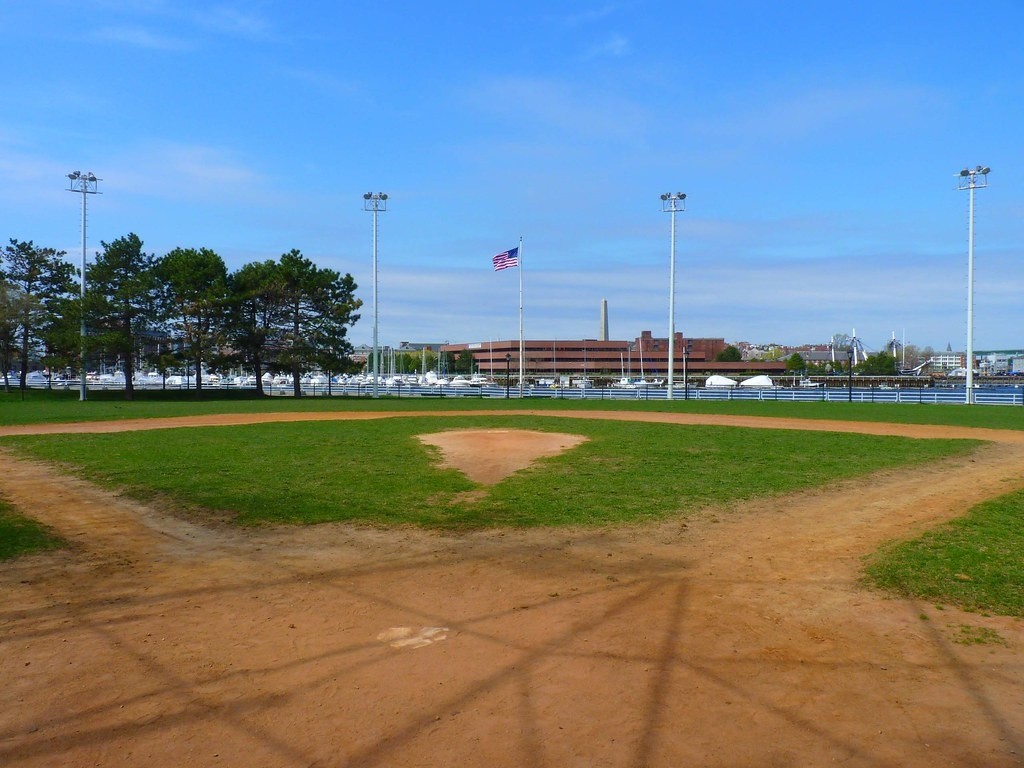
[330,375,337,383]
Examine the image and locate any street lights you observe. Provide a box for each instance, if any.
[955,165,994,405]
[661,192,688,400]
[68,169,103,402]
[362,189,387,398]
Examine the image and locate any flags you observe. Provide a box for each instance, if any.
[492,247,518,271]
